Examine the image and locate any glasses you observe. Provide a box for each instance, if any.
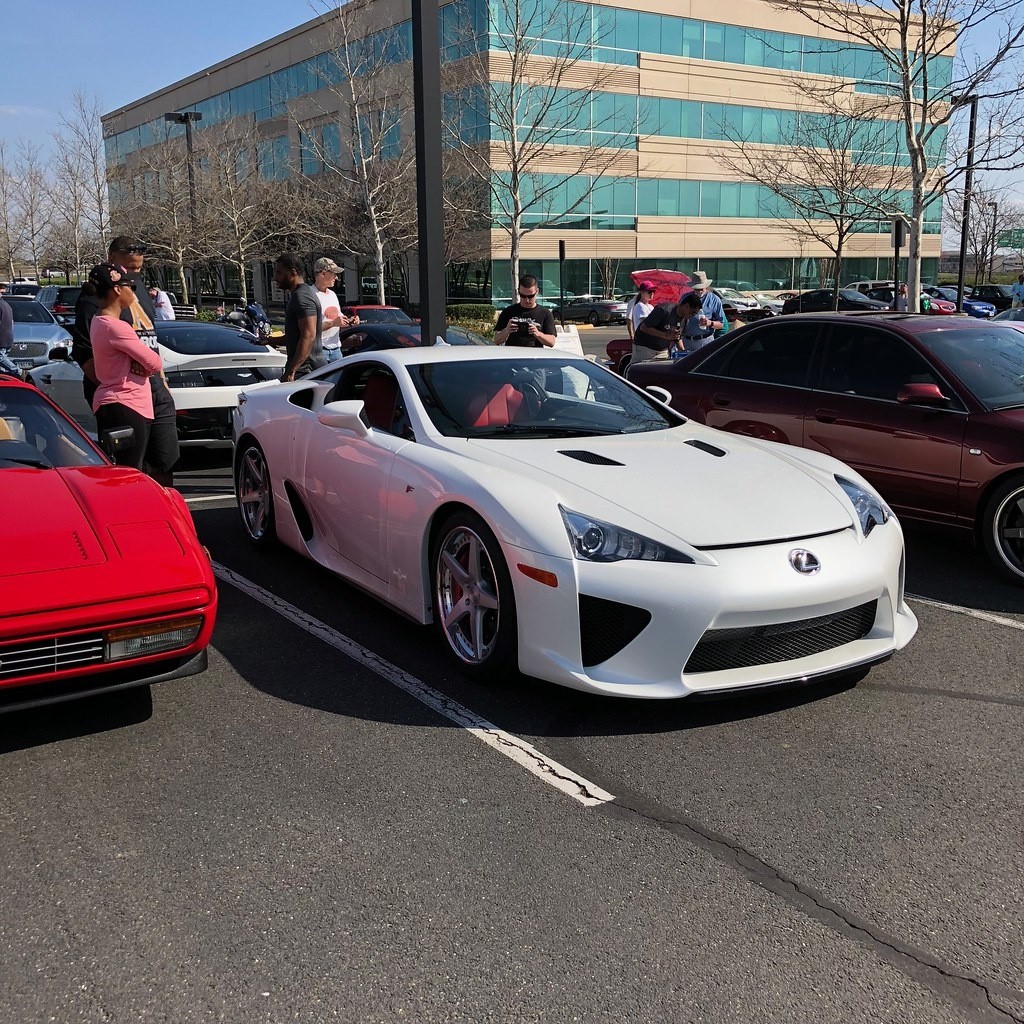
[643,290,656,294]
[113,282,137,292]
[518,291,537,298]
[108,244,148,255]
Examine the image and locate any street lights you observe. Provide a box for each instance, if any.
[949,93,980,313]
[164,110,203,314]
[987,202,997,282]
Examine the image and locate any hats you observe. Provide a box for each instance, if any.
[313,257,345,273]
[89,263,142,284]
[636,281,658,291]
[686,271,713,289]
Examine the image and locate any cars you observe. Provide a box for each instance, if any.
[553,271,1024,327]
[0,276,88,374]
[0,373,218,717]
[339,305,422,326]
[22,320,289,449]
[623,309,1024,594]
[336,323,500,356]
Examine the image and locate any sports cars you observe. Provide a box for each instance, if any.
[231,335,917,700]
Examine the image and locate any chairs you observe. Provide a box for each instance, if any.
[461,375,529,428]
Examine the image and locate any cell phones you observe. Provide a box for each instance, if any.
[513,321,529,331]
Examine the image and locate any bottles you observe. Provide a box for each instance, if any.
[674,322,680,343]
[698,309,707,330]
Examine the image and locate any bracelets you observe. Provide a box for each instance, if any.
[709,320,714,328]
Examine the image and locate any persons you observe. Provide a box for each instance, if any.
[706,286,729,340]
[147,287,177,348]
[920,284,930,312]
[630,291,702,364]
[626,288,640,339]
[632,281,660,335]
[677,271,724,351]
[0,294,28,379]
[309,257,360,366]
[1008,275,1024,320]
[889,285,908,312]
[82,262,162,473]
[278,263,322,383]
[71,236,180,486]
[494,274,557,390]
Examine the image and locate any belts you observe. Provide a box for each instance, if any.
[684,330,713,339]
[321,346,340,351]
